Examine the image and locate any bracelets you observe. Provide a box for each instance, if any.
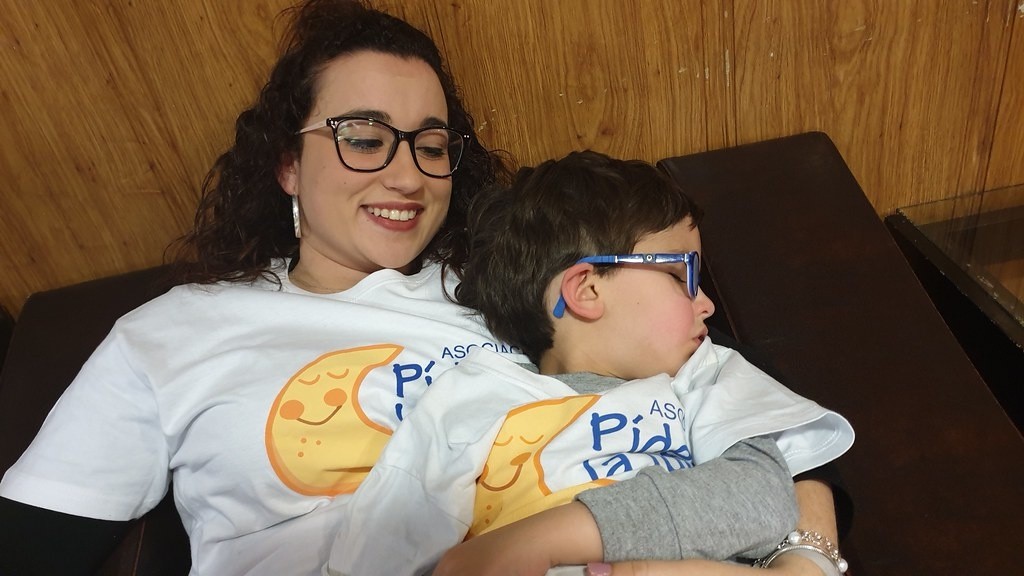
[749,528,848,576]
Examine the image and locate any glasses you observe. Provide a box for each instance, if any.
[294,115,472,180]
[553,251,701,319]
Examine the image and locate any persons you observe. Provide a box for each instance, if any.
[301,146,799,576]
[0,0,847,576]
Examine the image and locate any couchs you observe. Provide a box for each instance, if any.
[0,130,1024,576]
